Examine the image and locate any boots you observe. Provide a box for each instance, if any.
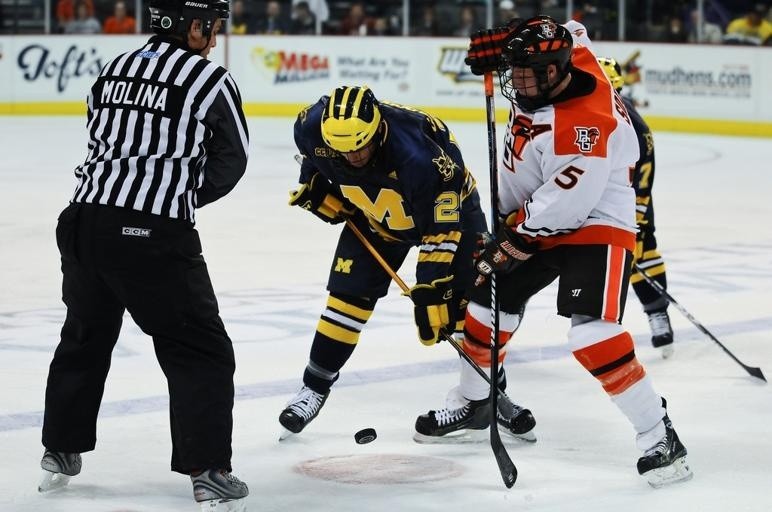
[278,384,331,433]
[637,395,688,476]
[644,303,675,346]
[414,394,494,437]
[495,387,536,434]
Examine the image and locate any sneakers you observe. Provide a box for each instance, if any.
[192,468,248,504]
[40,446,84,476]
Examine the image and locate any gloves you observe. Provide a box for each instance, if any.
[475,224,541,281]
[401,275,457,345]
[464,18,520,75]
[290,172,356,225]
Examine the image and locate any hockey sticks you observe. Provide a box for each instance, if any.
[484,72,517,490]
[637,266,765,380]
[294,153,515,420]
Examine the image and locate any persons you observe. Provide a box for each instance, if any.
[415,11,687,476]
[39,0,249,504]
[279,84,537,436]
[595,54,674,349]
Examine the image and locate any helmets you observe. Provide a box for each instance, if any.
[150,0,229,39]
[318,84,384,167]
[594,57,624,90]
[497,15,573,105]
